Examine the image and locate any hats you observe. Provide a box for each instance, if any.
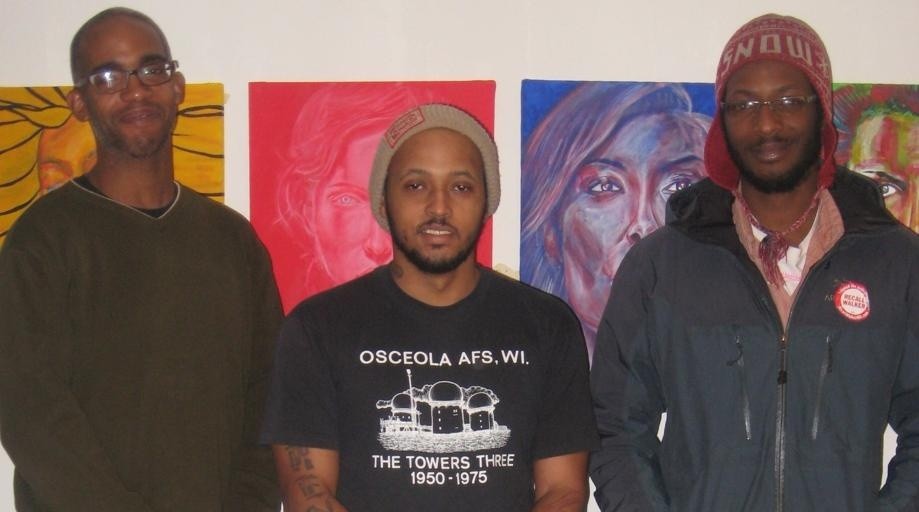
[369,104,500,232]
[705,14,838,192]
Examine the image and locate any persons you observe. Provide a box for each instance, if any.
[278,83,434,286]
[589,13,919,512]
[521,81,713,370]
[1,7,283,512]
[833,84,919,236]
[268,102,588,512]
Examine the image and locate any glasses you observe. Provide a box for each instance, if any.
[76,60,178,94]
[721,94,816,115]
